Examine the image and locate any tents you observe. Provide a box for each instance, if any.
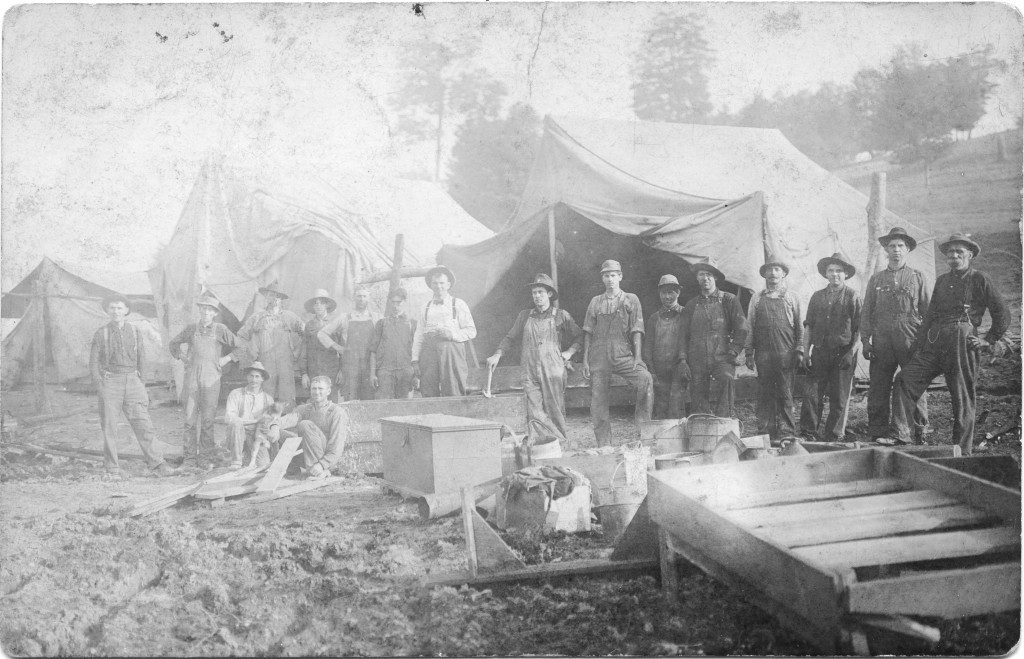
[1,115,935,404]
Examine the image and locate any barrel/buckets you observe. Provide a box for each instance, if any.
[743,447,780,461]
[639,418,688,455]
[519,435,562,458]
[654,452,704,470]
[710,430,747,456]
[595,460,648,548]
[685,414,744,453]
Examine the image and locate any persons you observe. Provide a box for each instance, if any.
[87,227,1011,485]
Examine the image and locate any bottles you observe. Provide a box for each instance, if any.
[781,438,810,455]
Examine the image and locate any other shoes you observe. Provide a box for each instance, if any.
[156,463,174,476]
[916,433,928,445]
[876,437,902,446]
[109,467,126,475]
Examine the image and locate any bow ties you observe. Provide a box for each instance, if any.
[434,300,444,306]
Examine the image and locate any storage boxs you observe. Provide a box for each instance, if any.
[378,415,502,495]
[495,465,593,533]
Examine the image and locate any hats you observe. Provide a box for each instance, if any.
[879,227,917,252]
[196,296,221,312]
[938,233,981,258]
[304,289,336,314]
[817,253,856,280]
[760,254,789,278]
[600,260,620,273]
[691,256,725,282]
[527,273,558,302]
[102,294,132,316]
[259,279,289,300]
[425,265,455,290]
[657,274,679,287]
[244,360,270,381]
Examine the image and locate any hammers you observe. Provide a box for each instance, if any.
[482,366,496,399]
